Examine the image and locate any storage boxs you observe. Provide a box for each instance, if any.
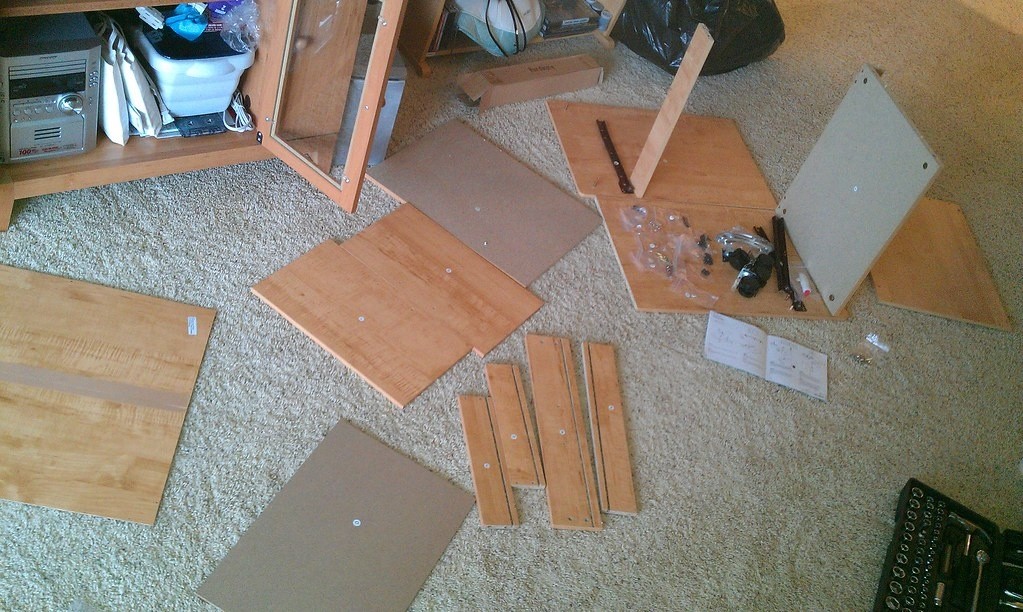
[451,53,606,117]
[129,28,256,116]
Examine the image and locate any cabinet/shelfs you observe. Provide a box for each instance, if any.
[0,0,405,223]
[399,0,627,90]
